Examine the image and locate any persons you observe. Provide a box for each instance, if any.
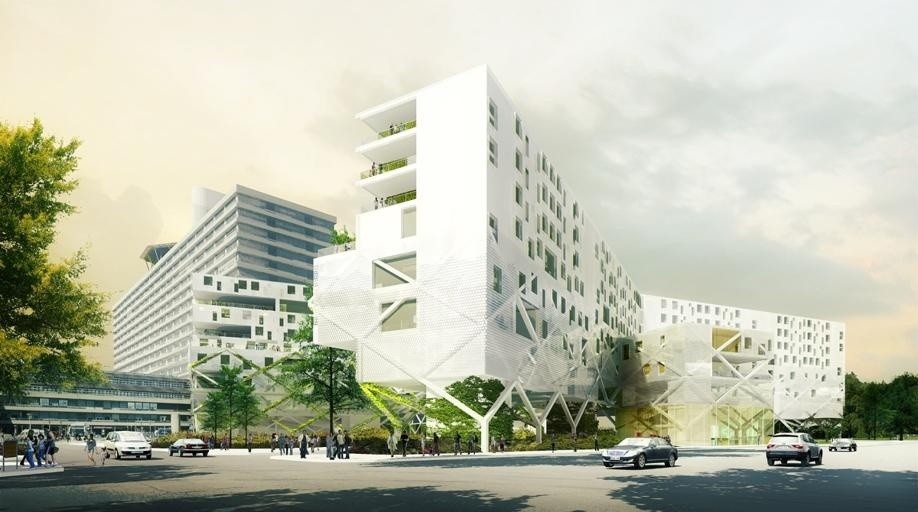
[594,432,599,451]
[271,430,352,460]
[400,431,409,457]
[370,162,383,177]
[389,122,406,135]
[199,429,230,451]
[500,433,505,451]
[454,431,463,455]
[19,426,108,469]
[551,432,557,453]
[471,432,478,455]
[247,434,253,452]
[432,432,440,456]
[374,196,388,209]
[387,431,398,458]
[492,436,496,453]
